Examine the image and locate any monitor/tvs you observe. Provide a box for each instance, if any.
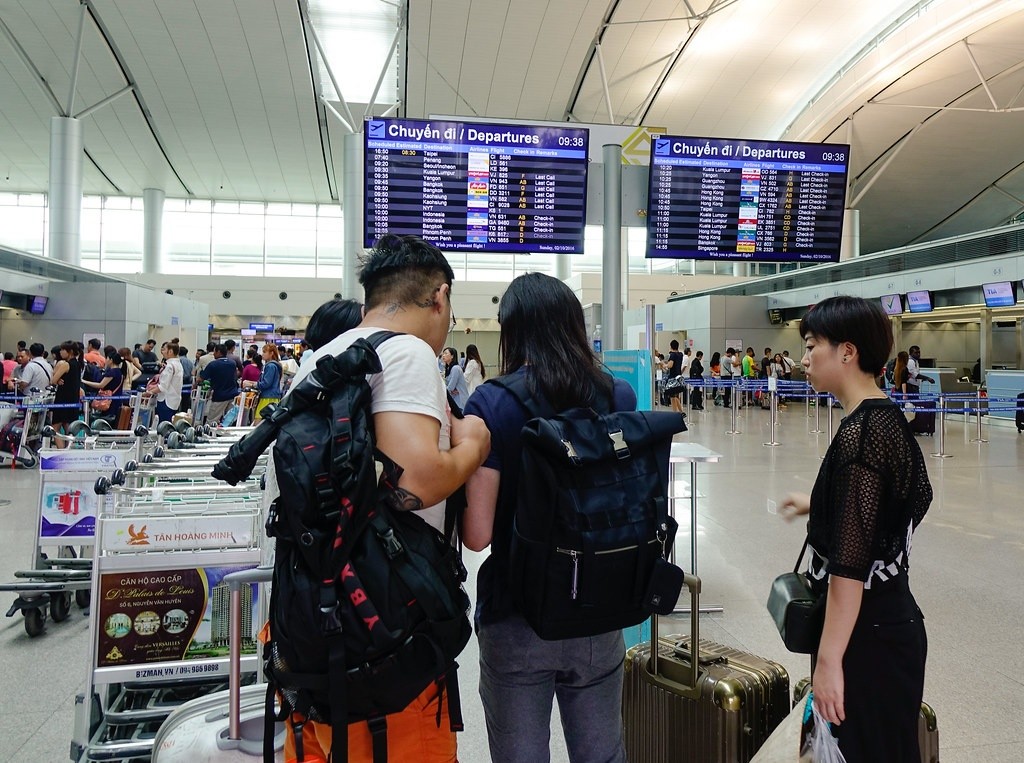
[31,296,49,315]
[363,117,589,255]
[645,135,850,265]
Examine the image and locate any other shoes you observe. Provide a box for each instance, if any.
[692,405,704,410]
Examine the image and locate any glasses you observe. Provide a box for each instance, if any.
[437,288,457,336]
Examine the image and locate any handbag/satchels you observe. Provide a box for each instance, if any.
[665,375,687,395]
[776,370,786,380]
[91,389,112,409]
[766,565,823,655]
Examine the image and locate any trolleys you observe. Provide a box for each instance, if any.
[0,386,56,468]
[1,416,279,763]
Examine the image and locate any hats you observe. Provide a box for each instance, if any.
[735,349,742,353]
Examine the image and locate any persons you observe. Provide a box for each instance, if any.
[973,359,981,384]
[894,345,935,435]
[263,233,492,763]
[437,345,487,418]
[655,340,796,418]
[779,295,932,763]
[0,299,364,448]
[462,273,636,763]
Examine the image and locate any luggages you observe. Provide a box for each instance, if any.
[795,676,940,763]
[914,399,936,436]
[0,419,29,453]
[624,571,789,763]
[150,565,287,763]
[1016,393,1024,433]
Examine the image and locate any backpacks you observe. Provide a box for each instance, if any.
[484,376,689,640]
[886,357,898,384]
[210,328,474,726]
